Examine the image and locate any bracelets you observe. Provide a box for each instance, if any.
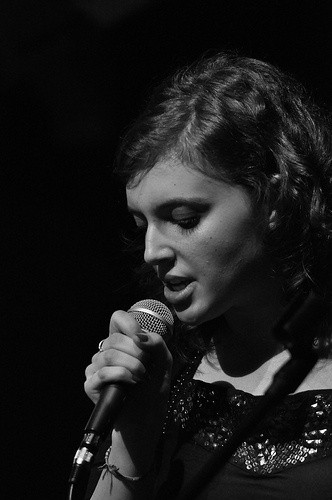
[97,445,139,482]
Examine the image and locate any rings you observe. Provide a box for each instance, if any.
[98,338,105,351]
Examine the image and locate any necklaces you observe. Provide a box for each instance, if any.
[161,337,203,442]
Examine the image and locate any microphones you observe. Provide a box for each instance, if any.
[69,299,174,488]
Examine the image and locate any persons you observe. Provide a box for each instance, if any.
[85,55,332,500]
